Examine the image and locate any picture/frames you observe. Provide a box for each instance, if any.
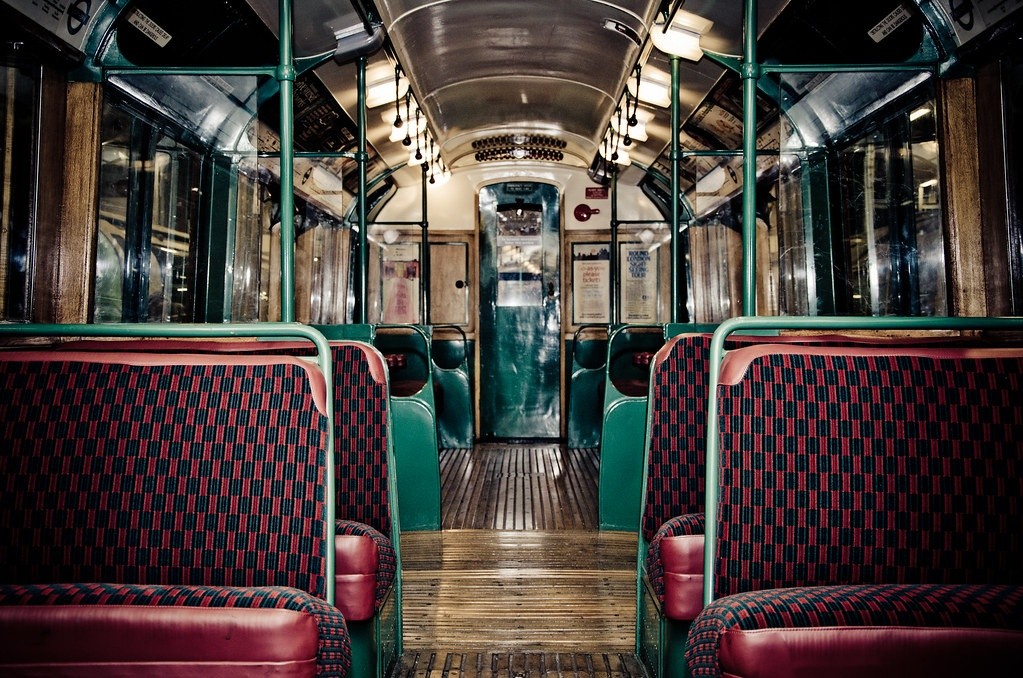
[571,240,613,326]
[619,241,661,326]
[378,240,423,324]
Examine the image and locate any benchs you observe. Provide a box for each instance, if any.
[2,316,1023,678]
